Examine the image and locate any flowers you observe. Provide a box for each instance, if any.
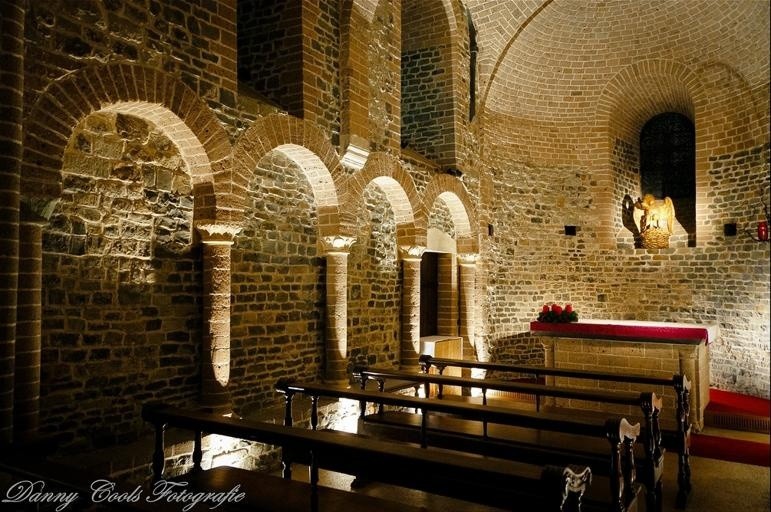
[537,304,578,323]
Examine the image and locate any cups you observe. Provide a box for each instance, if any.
[757,221,768,240]
